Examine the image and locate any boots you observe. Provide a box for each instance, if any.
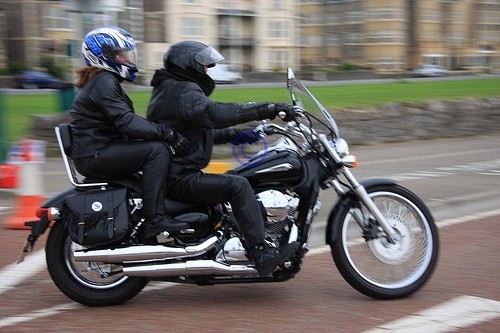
[244,241,300,279]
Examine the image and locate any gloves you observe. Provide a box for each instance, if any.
[166,128,191,158]
[275,102,296,122]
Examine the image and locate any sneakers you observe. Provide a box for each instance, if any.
[145,215,188,240]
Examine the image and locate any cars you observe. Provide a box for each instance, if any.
[15,71,61,90]
[414,64,447,78]
[207,65,245,83]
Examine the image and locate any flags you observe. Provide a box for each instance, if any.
[5,137,48,164]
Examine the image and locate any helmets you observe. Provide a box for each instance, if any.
[163,41,224,97]
[81,26,138,83]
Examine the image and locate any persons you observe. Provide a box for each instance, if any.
[145,40,300,277]
[66,26,188,232]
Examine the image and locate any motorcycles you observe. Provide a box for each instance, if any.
[18,66,440,307]
